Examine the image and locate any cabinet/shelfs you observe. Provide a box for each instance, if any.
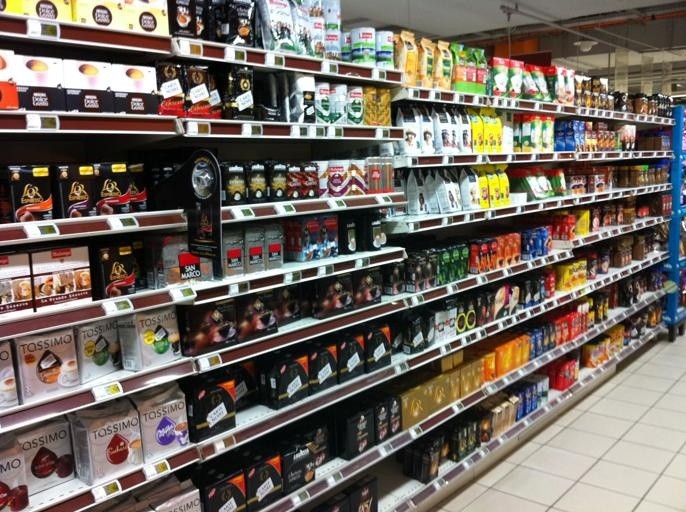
[0,1,686,512]
